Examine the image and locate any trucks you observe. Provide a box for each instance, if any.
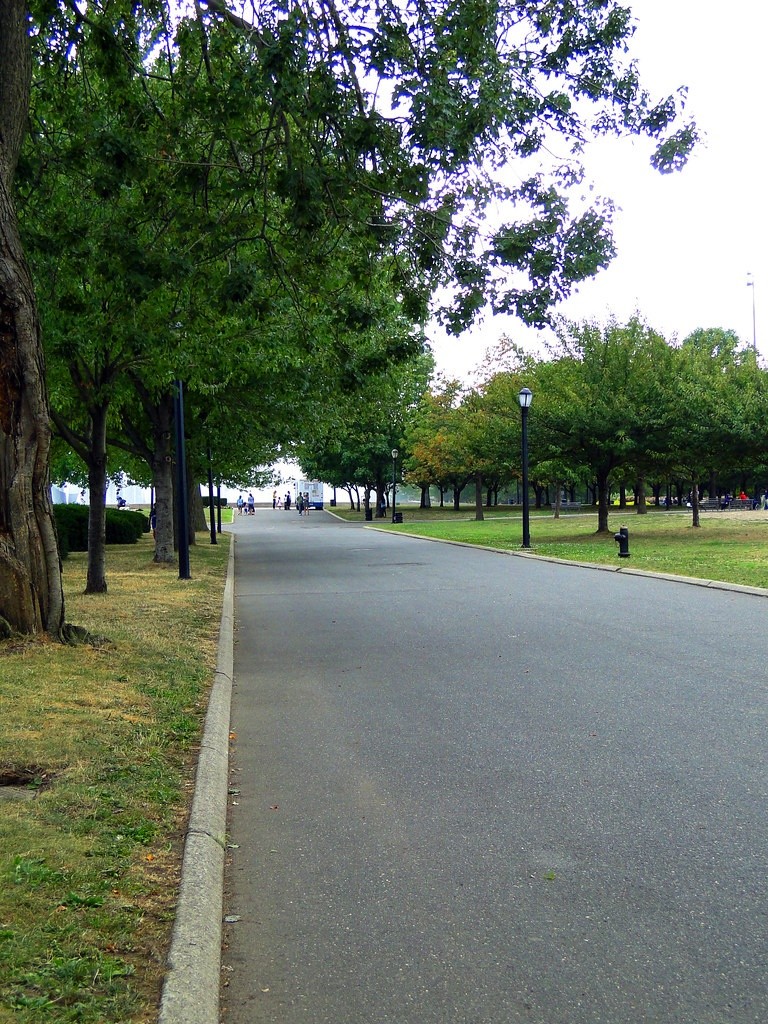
[294,478,324,510]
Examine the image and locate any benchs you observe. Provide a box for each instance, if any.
[724,499,754,512]
[551,502,586,515]
[699,498,722,511]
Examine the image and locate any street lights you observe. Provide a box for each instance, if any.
[171,321,193,580]
[518,387,537,551]
[390,448,399,525]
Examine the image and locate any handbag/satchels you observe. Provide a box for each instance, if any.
[238,501,243,506]
[381,503,386,509]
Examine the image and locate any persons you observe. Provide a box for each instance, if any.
[297,492,310,516]
[116,489,120,506]
[739,491,747,500]
[720,491,733,510]
[273,490,291,510]
[663,495,689,505]
[236,493,255,516]
[150,503,156,539]
[379,496,386,517]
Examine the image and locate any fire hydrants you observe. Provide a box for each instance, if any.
[614,524,631,558]
